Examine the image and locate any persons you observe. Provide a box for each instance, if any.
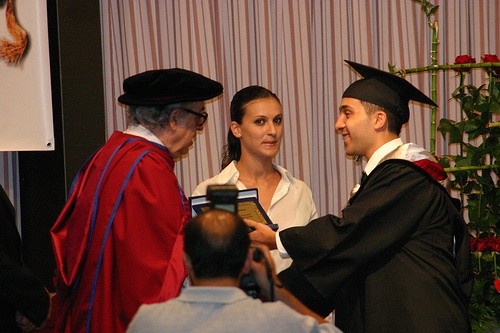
[0,185,50,333]
[191,86,320,273]
[244,60,473,333]
[38,68,224,333]
[127,209,343,333]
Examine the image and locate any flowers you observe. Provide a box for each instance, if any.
[432,53,500,327]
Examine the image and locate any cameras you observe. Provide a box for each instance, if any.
[206,184,261,264]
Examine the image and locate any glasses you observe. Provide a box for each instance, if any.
[178,106,208,126]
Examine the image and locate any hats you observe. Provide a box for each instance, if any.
[117,67,224,106]
[342,59,439,124]
